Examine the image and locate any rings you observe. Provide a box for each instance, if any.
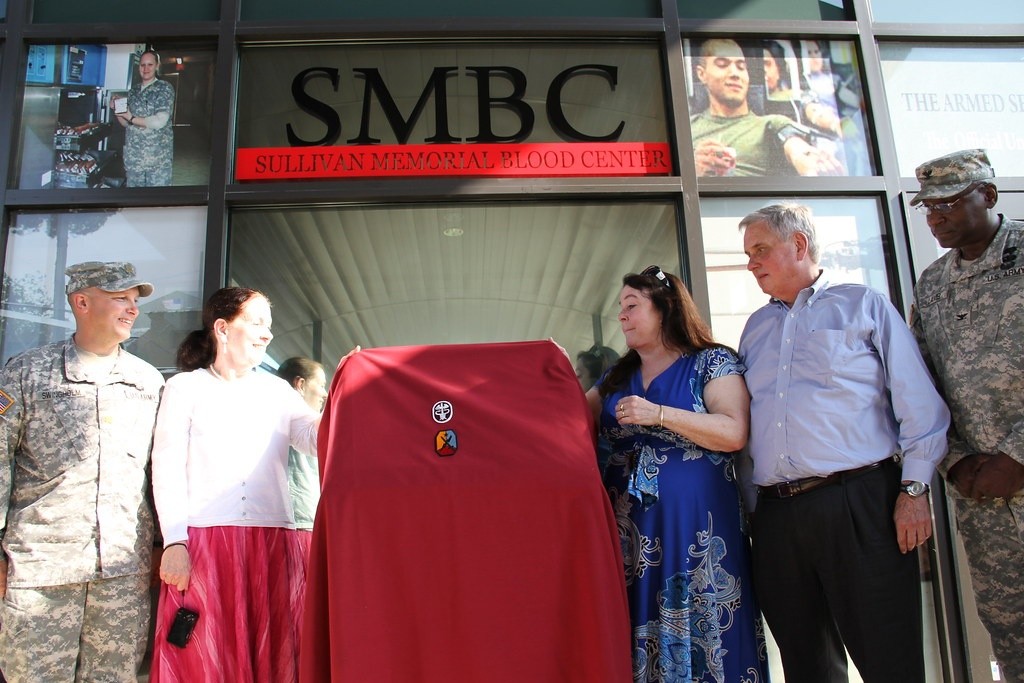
[619,404,623,410]
[621,410,624,417]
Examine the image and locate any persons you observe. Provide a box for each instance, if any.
[0,262,168,683]
[734,205,951,683]
[568,345,620,394]
[910,147,1024,683]
[689,37,864,179]
[150,286,361,683]
[274,357,328,578]
[546,265,772,683]
[109,51,176,186]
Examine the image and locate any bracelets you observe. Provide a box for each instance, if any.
[660,405,663,430]
[128,115,136,125]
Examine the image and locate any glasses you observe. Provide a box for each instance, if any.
[915,182,988,216]
[641,264,671,290]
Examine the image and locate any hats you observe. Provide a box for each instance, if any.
[64,260,154,297]
[910,147,997,208]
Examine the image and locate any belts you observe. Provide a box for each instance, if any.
[756,454,901,501]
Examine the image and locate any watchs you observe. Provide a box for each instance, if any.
[900,481,931,497]
